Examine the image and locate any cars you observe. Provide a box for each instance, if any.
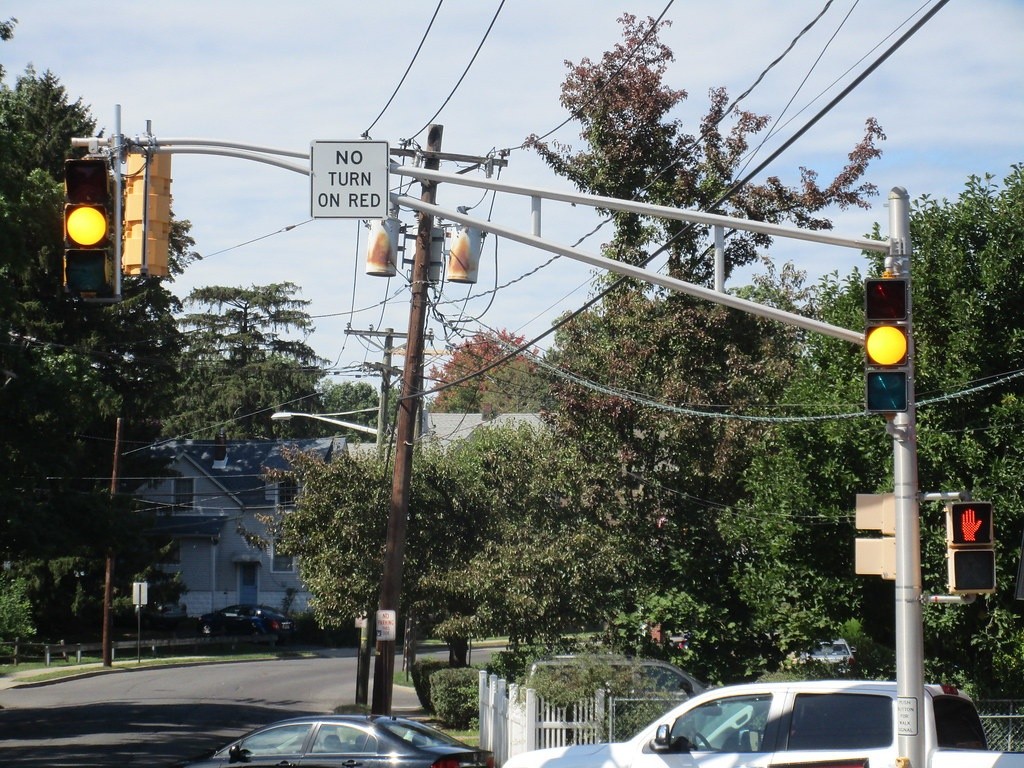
[529,655,710,714]
[198,603,297,644]
[175,713,496,768]
[108,593,187,631]
[789,637,862,679]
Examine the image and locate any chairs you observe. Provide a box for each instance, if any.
[356,735,377,752]
[323,735,340,752]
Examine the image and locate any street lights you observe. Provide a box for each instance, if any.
[268,324,394,706]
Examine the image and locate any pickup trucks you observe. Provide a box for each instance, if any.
[503,680,1024,765]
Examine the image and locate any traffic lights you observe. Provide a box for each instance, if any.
[65,158,113,303]
[950,502,995,594]
[863,273,912,415]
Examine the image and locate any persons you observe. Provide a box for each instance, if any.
[447,633,469,669]
